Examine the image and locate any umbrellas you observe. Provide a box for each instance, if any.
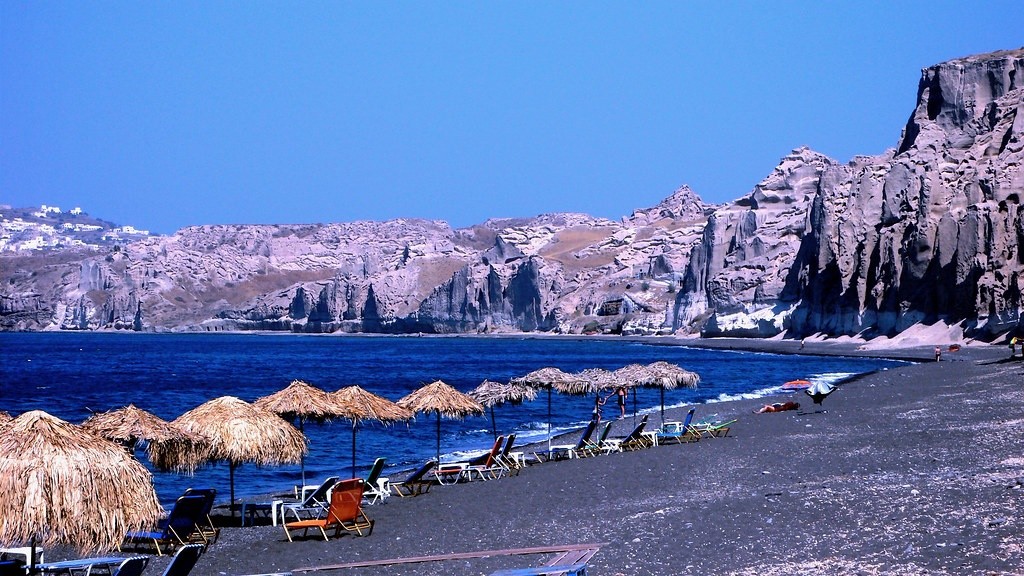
[806,380,838,404]
[0,360,700,576]
[781,379,812,412]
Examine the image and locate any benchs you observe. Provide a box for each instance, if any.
[537,548,599,576]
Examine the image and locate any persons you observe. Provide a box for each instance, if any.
[1021,339,1024,356]
[606,387,626,420]
[753,402,800,413]
[592,396,607,431]
[1009,337,1018,356]
[935,346,941,362]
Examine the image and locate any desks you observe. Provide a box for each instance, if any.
[438,462,472,483]
[661,421,684,433]
[302,484,335,504]
[507,452,526,469]
[0,408,740,576]
[549,443,576,459]
[363,478,391,499]
[0,547,45,576]
[604,440,624,453]
[640,431,659,447]
